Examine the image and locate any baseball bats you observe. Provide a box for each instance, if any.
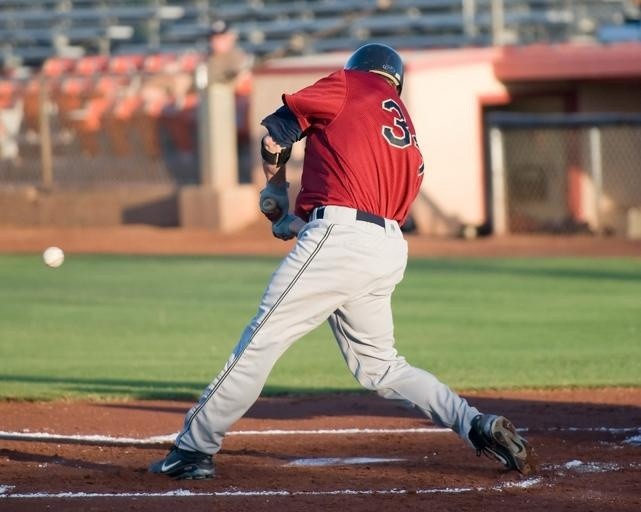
[262,198,280,221]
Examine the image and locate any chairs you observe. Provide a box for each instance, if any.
[0,0,640,43]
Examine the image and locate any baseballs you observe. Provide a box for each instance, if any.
[44,247,64,268]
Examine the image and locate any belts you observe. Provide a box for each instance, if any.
[308,205,386,229]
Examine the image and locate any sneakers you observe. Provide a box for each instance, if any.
[147,446,216,481]
[469,412,540,475]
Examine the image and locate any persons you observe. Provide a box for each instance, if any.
[563,160,598,233]
[146,42,536,479]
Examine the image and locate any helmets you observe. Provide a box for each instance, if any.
[344,43,404,97]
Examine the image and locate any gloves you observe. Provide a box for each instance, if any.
[271,214,297,241]
[258,181,290,223]
